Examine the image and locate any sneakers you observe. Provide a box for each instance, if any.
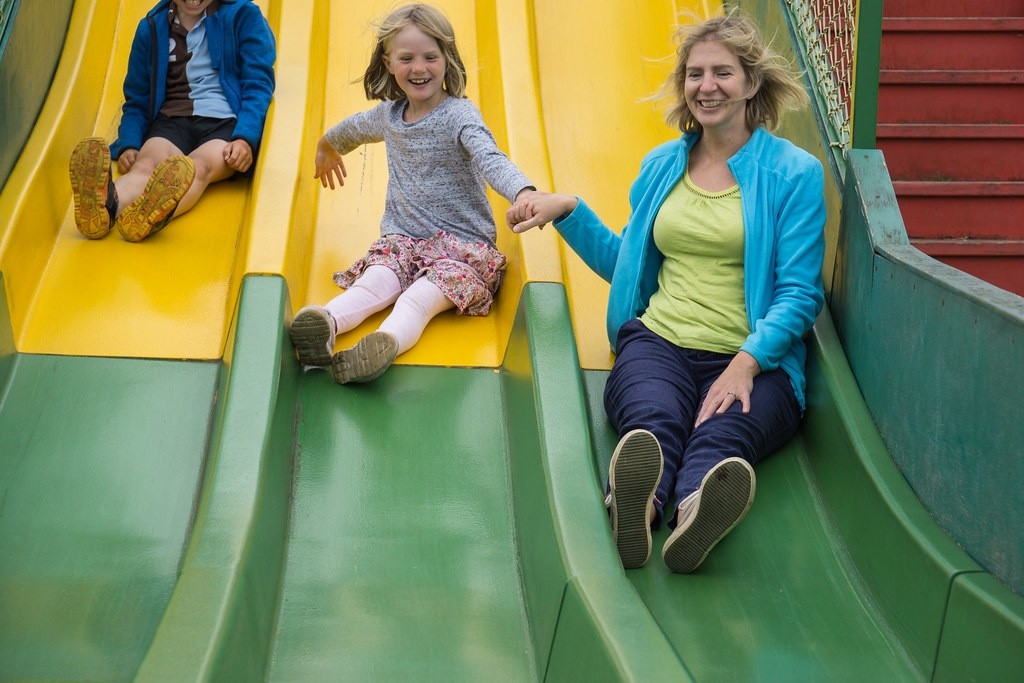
[663,457,756,574]
[604,429,664,570]
[333,332,399,385]
[290,307,336,367]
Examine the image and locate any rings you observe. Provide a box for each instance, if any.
[726,392,736,400]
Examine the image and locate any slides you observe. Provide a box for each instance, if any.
[0,0,1024,683]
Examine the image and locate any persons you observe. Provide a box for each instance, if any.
[69,0,277,242]
[505,3,828,574]
[290,3,548,386]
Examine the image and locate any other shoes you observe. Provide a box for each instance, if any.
[69,137,119,240]
[117,156,196,243]
